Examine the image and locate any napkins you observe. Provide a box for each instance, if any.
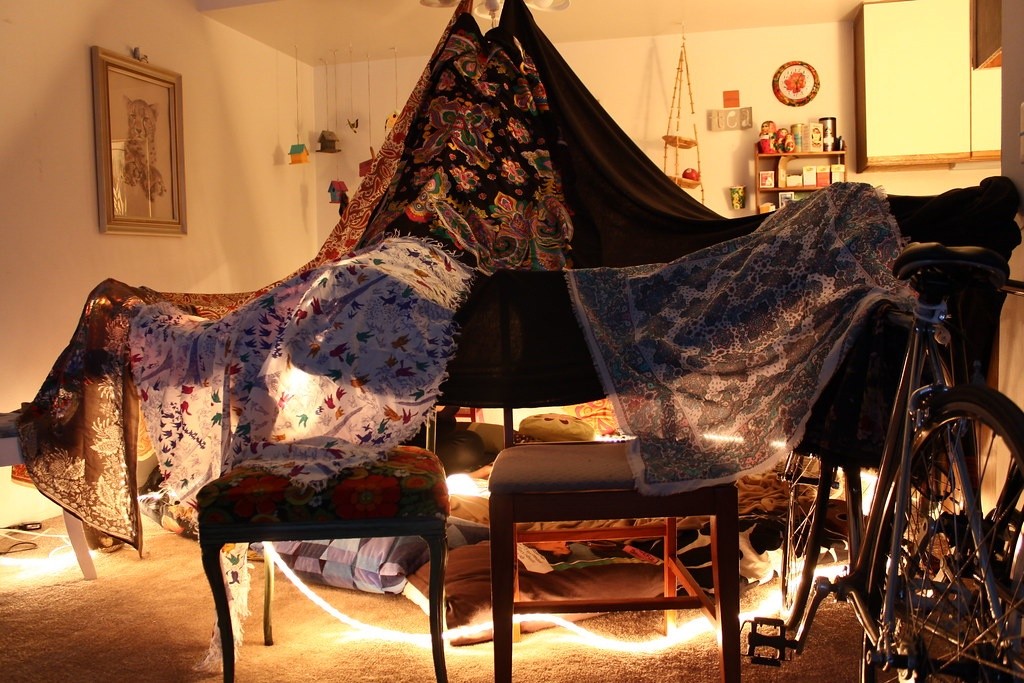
[759,202,776,215]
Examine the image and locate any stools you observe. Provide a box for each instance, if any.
[488,439,741,683]
[0,412,98,581]
[196,445,447,683]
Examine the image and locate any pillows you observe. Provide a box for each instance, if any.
[137,493,198,542]
[249,535,430,595]
[402,538,665,649]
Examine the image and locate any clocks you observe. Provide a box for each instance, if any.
[772,60,820,107]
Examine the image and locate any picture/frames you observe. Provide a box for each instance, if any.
[91,44,188,238]
[759,170,774,188]
[778,191,795,209]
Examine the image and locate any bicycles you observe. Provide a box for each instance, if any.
[746,238,1023,683]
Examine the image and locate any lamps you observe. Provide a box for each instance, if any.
[419,0,571,29]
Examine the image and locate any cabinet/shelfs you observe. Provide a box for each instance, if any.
[754,142,847,216]
[663,22,705,207]
[853,1,1002,174]
